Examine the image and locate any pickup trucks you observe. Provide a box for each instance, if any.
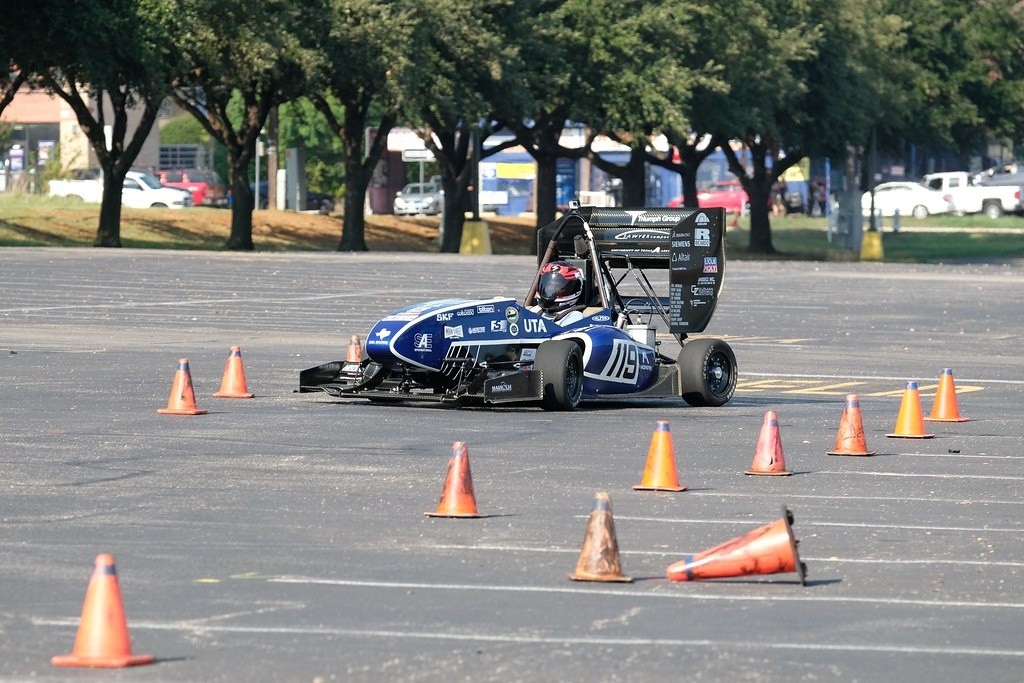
[50,171,193,208]
[920,172,1022,219]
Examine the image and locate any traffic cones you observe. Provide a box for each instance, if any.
[825,393,876,457]
[211,346,255,399]
[744,410,794,476]
[424,440,496,517]
[665,505,806,586]
[566,491,633,581]
[631,421,689,492]
[346,333,362,362]
[923,368,971,423]
[884,382,936,439]
[49,553,155,668]
[155,358,208,415]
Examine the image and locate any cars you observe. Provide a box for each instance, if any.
[152,170,228,207]
[668,181,773,213]
[392,183,445,216]
[972,161,1024,208]
[834,182,957,219]
[228,181,336,211]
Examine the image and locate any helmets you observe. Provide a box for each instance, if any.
[533,261,585,314]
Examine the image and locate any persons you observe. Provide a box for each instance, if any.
[807,180,826,217]
[777,176,786,201]
[528,261,584,327]
